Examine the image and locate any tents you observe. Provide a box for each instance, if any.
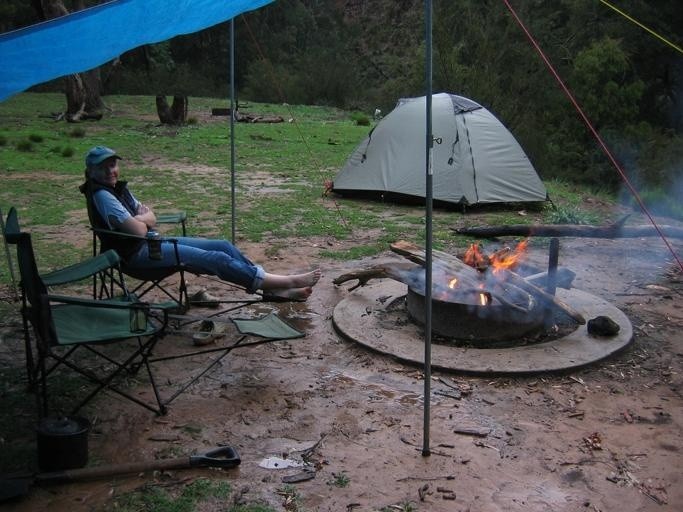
[327,91,548,213]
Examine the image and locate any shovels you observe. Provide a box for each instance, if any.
[0,445,241,503]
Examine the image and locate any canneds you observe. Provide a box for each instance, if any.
[148,231,159,240]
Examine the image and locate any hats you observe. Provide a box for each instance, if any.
[85,146,122,165]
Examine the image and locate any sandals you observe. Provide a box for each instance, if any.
[187,290,220,308]
[192,319,225,346]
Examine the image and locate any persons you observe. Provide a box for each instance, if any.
[76,145,322,303]
